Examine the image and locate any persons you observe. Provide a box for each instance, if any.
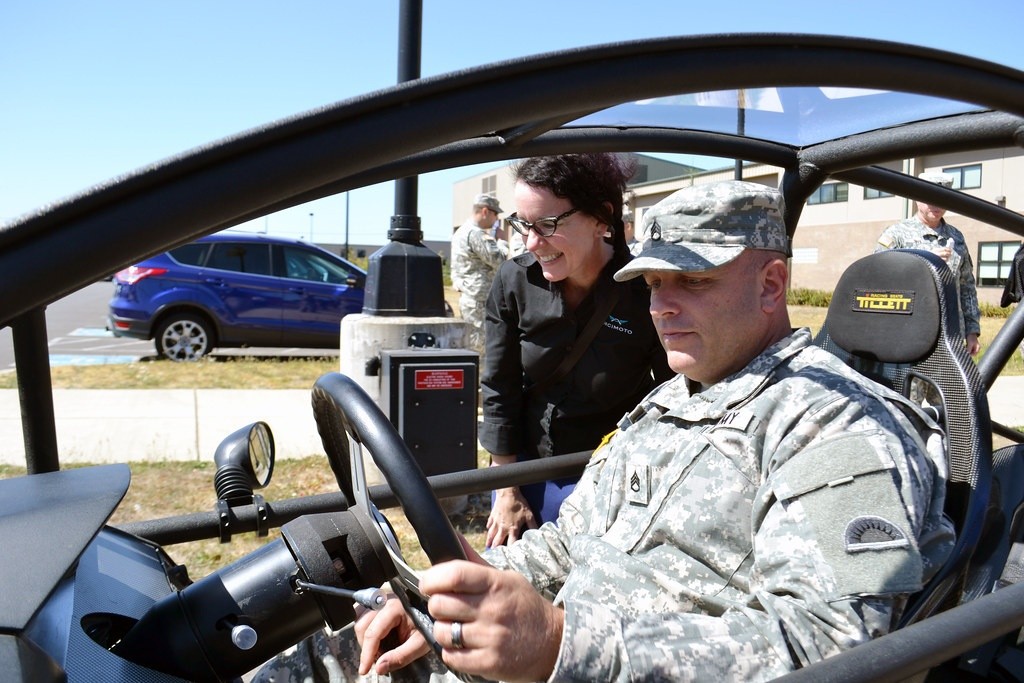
[352,151,946,683]
[874,172,980,355]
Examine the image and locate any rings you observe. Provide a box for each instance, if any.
[451,622,465,649]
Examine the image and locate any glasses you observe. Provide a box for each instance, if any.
[504,209,581,238]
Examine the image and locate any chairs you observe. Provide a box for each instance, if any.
[812,249,995,634]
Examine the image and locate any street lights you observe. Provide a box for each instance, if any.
[311,212,315,244]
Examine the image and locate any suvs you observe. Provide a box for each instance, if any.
[105,232,456,368]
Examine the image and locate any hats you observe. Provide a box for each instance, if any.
[613,180,790,282]
[918,172,955,190]
[472,193,503,214]
[620,211,635,223]
